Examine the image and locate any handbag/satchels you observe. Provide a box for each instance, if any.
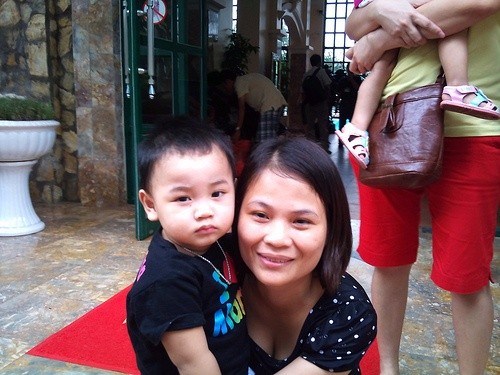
[358,82,445,189]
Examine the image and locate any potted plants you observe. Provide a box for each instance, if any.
[0,93,62,237]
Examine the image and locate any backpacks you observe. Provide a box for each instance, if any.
[301,68,326,106]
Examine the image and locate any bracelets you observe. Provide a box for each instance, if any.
[235,127,240,131]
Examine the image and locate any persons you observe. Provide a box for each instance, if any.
[332,0,500,171]
[300,54,364,154]
[217,69,288,149]
[125,114,252,375]
[346,1,500,375]
[230,132,378,375]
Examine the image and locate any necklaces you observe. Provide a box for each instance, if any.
[162,229,231,286]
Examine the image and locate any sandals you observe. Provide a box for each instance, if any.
[439,84,500,120]
[334,119,370,170]
